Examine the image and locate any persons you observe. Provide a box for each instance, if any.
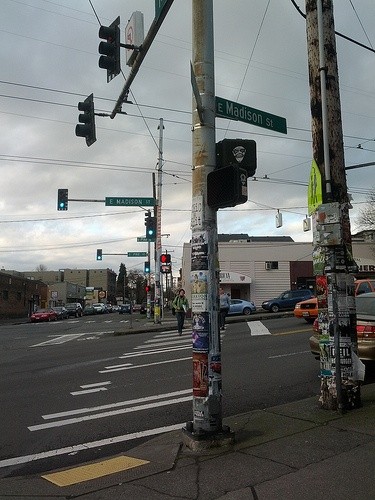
[172,289,189,336]
[220,287,230,329]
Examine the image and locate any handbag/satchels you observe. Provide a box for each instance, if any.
[172,307,175,315]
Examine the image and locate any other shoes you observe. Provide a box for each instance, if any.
[221,327,225,330]
[178,332,182,336]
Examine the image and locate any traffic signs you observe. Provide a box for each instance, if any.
[191,59,205,125]
[216,96,289,136]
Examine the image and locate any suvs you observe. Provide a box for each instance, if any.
[64,302,83,317]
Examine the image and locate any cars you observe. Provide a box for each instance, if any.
[105,304,119,312]
[133,304,141,312]
[31,307,57,322]
[118,304,132,314]
[221,298,256,316]
[140,301,154,314]
[82,305,97,314]
[51,307,69,319]
[293,279,375,322]
[262,289,316,313]
[309,291,375,363]
[91,303,105,314]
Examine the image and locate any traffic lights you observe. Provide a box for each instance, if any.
[160,265,171,273]
[75,93,97,147]
[146,285,150,291]
[58,188,68,211]
[98,16,122,84]
[145,212,150,225]
[146,217,156,239]
[97,248,102,260]
[159,253,171,263]
[145,261,150,272]
[206,164,249,210]
